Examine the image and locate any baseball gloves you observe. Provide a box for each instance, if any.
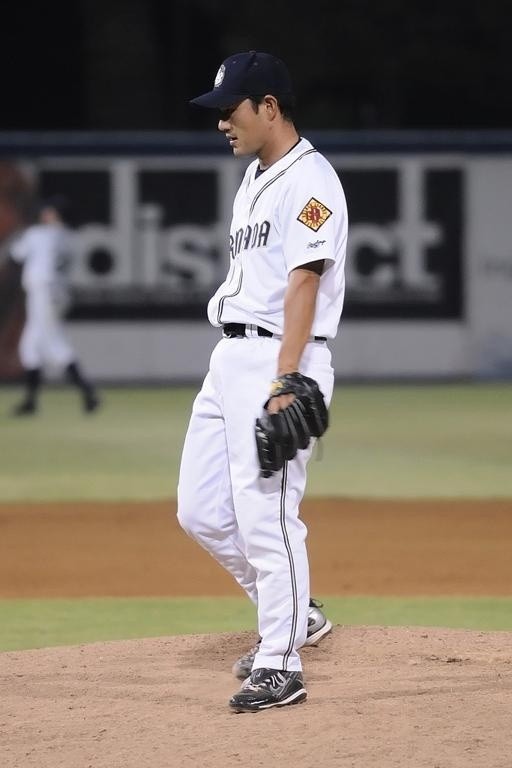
[254,372,329,479]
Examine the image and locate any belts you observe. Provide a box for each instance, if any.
[220,319,327,340]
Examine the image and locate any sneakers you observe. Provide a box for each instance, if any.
[234,599,334,677]
[17,401,34,414]
[228,673,306,714]
[87,397,93,410]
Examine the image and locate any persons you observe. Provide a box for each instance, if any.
[5,203,102,415]
[176,53,351,711]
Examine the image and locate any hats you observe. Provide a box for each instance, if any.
[187,49,298,107]
